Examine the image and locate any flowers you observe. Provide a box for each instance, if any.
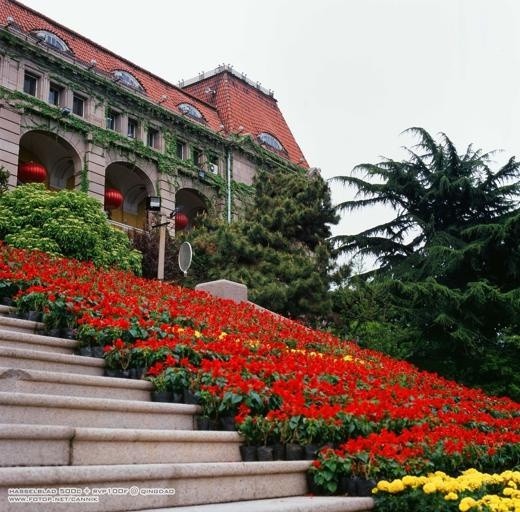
[0,245,520,512]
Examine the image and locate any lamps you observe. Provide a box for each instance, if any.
[194,168,205,180]
[157,95,168,104]
[204,86,216,94]
[112,72,123,83]
[87,60,97,70]
[4,16,15,28]
[35,33,47,44]
[59,107,71,116]
[216,124,225,134]
[145,196,161,211]
[296,158,305,165]
[236,125,244,135]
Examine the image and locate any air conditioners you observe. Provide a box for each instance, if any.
[208,162,218,174]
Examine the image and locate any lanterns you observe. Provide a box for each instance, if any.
[176,212,189,230]
[105,187,125,209]
[18,160,48,183]
